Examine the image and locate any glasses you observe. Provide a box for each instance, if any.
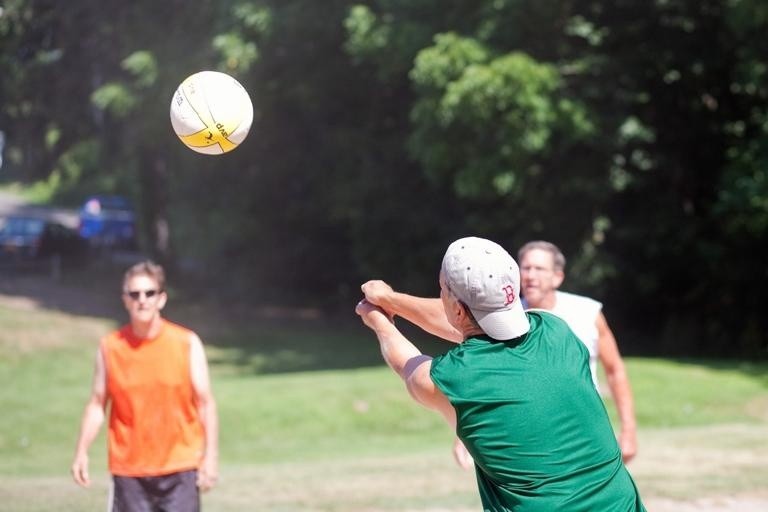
[124,288,160,300]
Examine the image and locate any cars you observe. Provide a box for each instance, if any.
[0,195,136,266]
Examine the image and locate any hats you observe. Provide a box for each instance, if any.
[440,236,530,341]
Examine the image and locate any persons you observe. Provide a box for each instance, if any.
[452,241,640,473]
[354,235,647,512]
[70,260,219,511]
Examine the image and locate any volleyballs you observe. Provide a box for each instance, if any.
[169,70,253,156]
